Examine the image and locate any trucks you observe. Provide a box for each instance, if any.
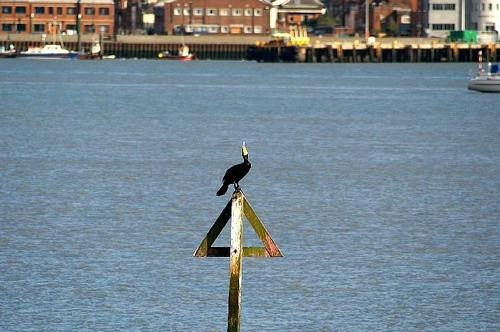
[445,30,478,45]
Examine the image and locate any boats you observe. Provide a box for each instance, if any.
[19,44,80,59]
[0,50,17,58]
[158,53,195,60]
[79,54,116,60]
[467,72,500,92]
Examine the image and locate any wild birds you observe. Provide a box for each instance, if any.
[217,140,252,197]
